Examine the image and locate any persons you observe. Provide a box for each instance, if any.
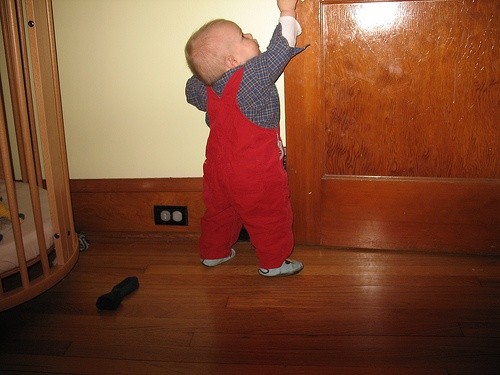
[185,0,310,277]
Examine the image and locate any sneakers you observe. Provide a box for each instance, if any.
[259,259,303,278]
[200,247,236,267]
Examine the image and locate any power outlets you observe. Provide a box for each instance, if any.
[153,205,188,226]
